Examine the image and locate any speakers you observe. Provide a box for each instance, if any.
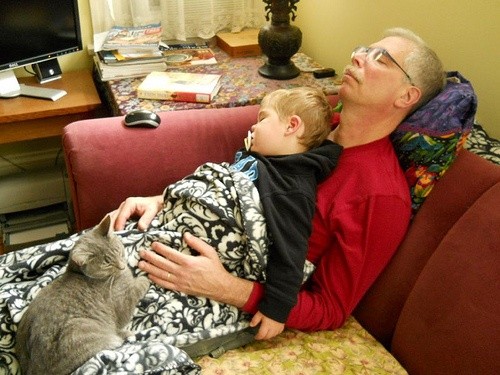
[34,58,63,84]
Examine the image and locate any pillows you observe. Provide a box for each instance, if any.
[388,70,480,219]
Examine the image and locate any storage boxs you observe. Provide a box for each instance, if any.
[215,27,263,57]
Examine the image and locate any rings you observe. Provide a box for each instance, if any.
[166,272,170,281]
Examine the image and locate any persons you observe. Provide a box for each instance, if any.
[113,27,445,332]
[230,86,342,340]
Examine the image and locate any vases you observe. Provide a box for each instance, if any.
[258,0,301,81]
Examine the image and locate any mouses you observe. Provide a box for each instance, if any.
[124,110,161,128]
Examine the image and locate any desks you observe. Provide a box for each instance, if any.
[93,40,342,123]
[0,69,103,253]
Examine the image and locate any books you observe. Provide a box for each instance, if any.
[93,22,222,104]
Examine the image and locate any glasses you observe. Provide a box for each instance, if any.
[350,46,415,87]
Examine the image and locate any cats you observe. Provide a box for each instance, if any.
[15,214,151,375]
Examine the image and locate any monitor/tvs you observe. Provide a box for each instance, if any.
[0,0,84,101]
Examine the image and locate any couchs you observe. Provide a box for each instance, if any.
[61,92,500,375]
